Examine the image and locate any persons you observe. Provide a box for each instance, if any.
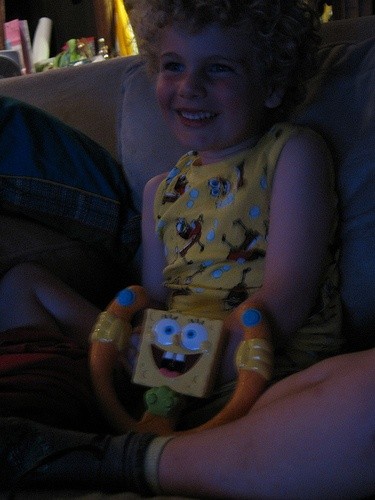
[0,0,375,500]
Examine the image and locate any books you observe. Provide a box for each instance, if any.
[4,19,35,76]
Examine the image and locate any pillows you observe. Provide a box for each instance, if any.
[0,94,141,261]
[1,213,103,303]
[117,37,375,352]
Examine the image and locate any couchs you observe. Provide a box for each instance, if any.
[0,14,375,500]
